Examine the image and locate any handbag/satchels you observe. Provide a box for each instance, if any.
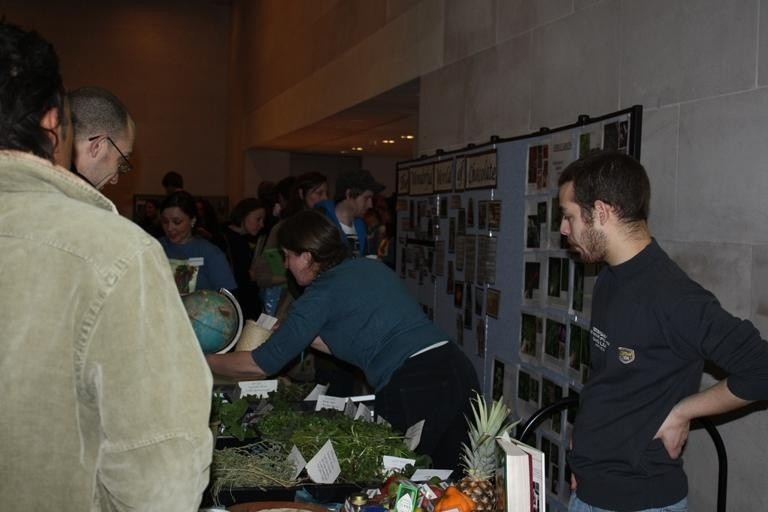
[273,287,316,382]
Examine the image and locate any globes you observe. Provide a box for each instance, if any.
[183,288,244,354]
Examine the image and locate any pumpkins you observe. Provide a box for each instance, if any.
[435,485,476,512]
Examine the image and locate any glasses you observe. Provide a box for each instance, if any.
[88,134,133,174]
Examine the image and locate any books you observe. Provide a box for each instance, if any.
[493,435,547,512]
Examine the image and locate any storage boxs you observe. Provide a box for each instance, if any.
[211,437,382,506]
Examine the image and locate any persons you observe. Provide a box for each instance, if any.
[151,163,395,399]
[558,151,768,511]
[68,84,135,195]
[0,18,218,512]
[205,209,480,484]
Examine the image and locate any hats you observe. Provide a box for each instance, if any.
[337,169,386,195]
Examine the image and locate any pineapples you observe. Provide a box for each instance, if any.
[461,390,523,512]
